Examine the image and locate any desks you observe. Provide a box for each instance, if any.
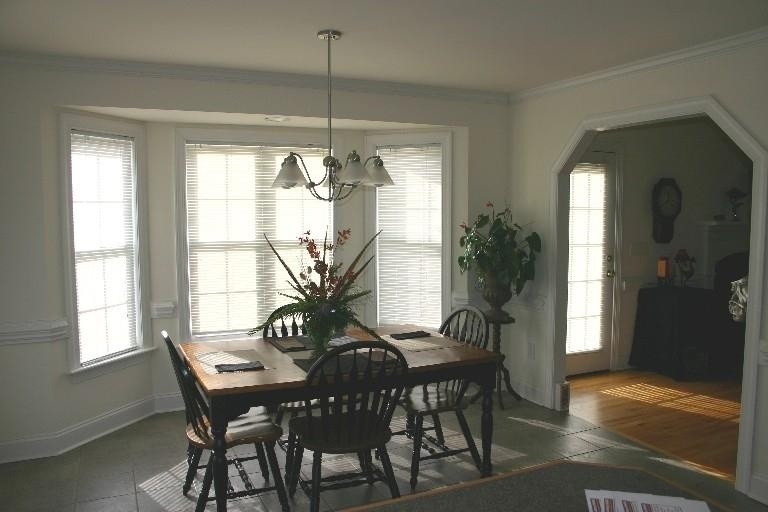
[178,324,497,511]
[470,318,520,409]
[626,287,730,382]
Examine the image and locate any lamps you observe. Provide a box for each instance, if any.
[269,28,394,202]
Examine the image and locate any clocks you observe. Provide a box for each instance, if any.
[651,177,681,244]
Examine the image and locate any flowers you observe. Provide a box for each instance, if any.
[458,200,539,296]
[242,225,388,349]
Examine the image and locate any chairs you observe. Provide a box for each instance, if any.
[283,341,410,511]
[262,303,344,426]
[159,329,289,511]
[375,305,489,490]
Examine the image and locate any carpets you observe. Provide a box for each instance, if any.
[339,458,732,512]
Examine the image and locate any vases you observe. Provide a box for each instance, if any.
[478,273,514,320]
[305,318,335,355]
